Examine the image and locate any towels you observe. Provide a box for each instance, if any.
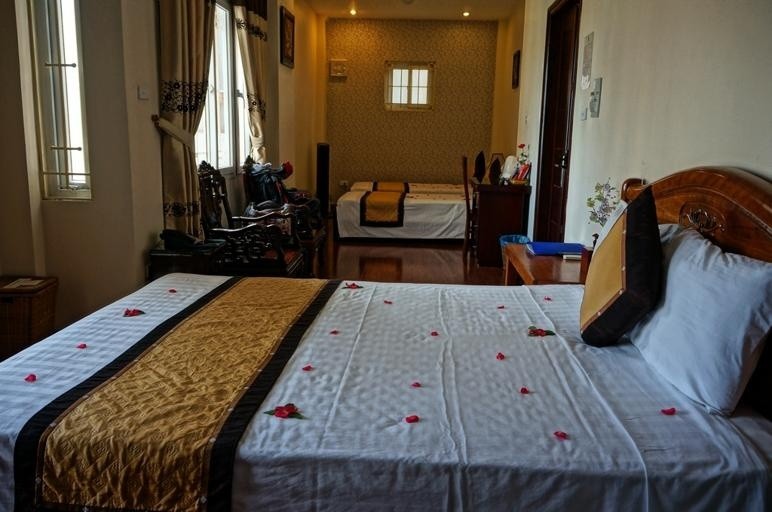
[499,235,529,271]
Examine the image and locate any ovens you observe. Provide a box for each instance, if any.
[335,179,474,240]
[2,162,771,510]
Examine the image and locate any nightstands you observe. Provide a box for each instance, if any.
[500,241,589,285]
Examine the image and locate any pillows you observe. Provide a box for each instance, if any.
[593,199,679,246]
[579,184,664,348]
[631,229,772,420]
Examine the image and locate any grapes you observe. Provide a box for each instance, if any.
[500,241,589,285]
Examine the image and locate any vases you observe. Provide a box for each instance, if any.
[515,143,528,164]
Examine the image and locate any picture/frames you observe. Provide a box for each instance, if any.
[279,5,295,69]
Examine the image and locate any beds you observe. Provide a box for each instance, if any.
[2,162,771,510]
[335,179,474,240]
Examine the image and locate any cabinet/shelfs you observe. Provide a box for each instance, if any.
[471,175,532,263]
[146,238,227,283]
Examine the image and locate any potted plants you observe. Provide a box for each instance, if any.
[279,5,295,69]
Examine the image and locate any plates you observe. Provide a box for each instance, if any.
[146,238,227,283]
[471,175,532,263]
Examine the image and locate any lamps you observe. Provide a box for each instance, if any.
[460,153,475,259]
[198,155,326,277]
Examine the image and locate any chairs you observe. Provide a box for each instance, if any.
[198,155,326,277]
[460,153,475,259]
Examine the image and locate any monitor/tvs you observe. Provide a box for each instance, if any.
[526,242,583,255]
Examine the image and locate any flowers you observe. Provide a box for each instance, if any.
[515,143,528,164]
